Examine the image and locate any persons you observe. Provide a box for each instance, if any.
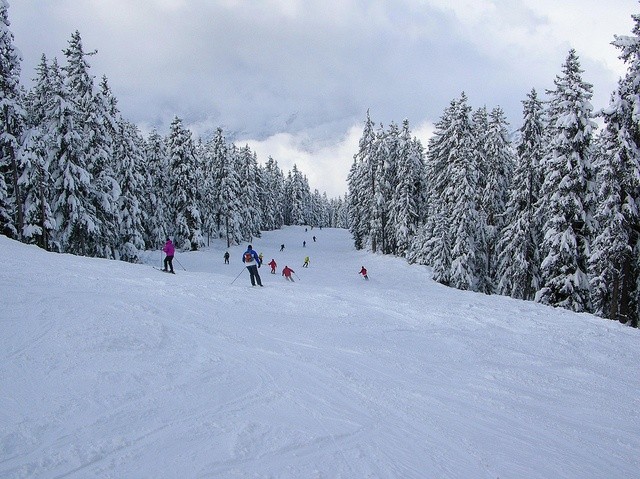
[163,238,174,272]
[280,244,285,252]
[282,266,294,277]
[303,241,306,248]
[305,228,307,232]
[224,252,229,264]
[358,266,368,279]
[303,256,310,268]
[242,245,262,285]
[313,236,316,242]
[268,259,277,274]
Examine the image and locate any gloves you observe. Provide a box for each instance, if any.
[258,264,260,268]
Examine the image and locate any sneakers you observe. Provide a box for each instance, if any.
[162,268,167,271]
[252,284,256,286]
[259,284,263,287]
[169,270,174,273]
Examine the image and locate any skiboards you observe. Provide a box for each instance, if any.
[152,266,176,275]
